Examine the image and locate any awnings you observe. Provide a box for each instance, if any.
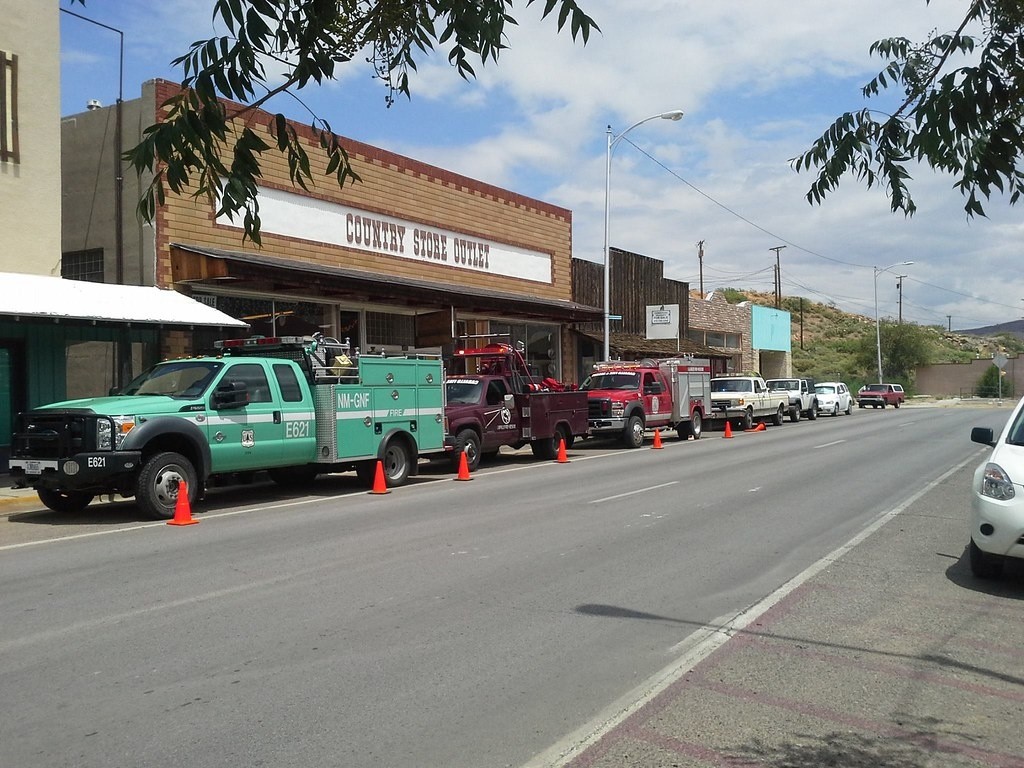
[581,331,732,359]
[170,243,604,322]
[0,272,251,328]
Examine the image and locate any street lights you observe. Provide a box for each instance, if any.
[873,261,913,383]
[603,110,683,362]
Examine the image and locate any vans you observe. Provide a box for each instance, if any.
[812,382,853,416]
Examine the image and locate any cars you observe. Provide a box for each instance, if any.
[970,396,1024,560]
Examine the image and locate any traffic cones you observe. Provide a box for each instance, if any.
[650,428,665,449]
[367,461,391,495]
[755,421,766,431]
[722,421,734,438]
[554,439,572,463]
[167,481,199,526]
[453,452,474,482]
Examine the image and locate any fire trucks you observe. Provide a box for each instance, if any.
[9,332,459,521]
[580,353,713,449]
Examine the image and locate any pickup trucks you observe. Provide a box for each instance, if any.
[429,343,589,470]
[765,378,818,423]
[857,384,904,409]
[702,376,789,429]
